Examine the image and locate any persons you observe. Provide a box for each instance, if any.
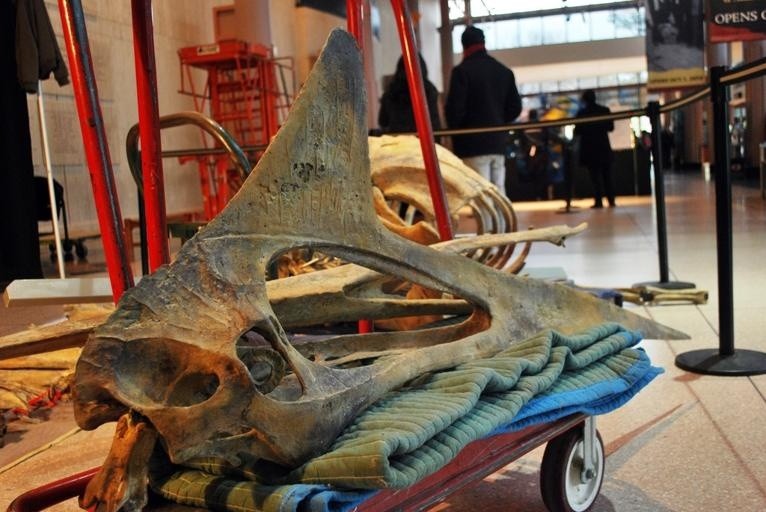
[572,89,616,208]
[443,26,522,233]
[378,50,441,225]
[519,109,552,201]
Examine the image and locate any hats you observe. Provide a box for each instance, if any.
[461,26,485,46]
